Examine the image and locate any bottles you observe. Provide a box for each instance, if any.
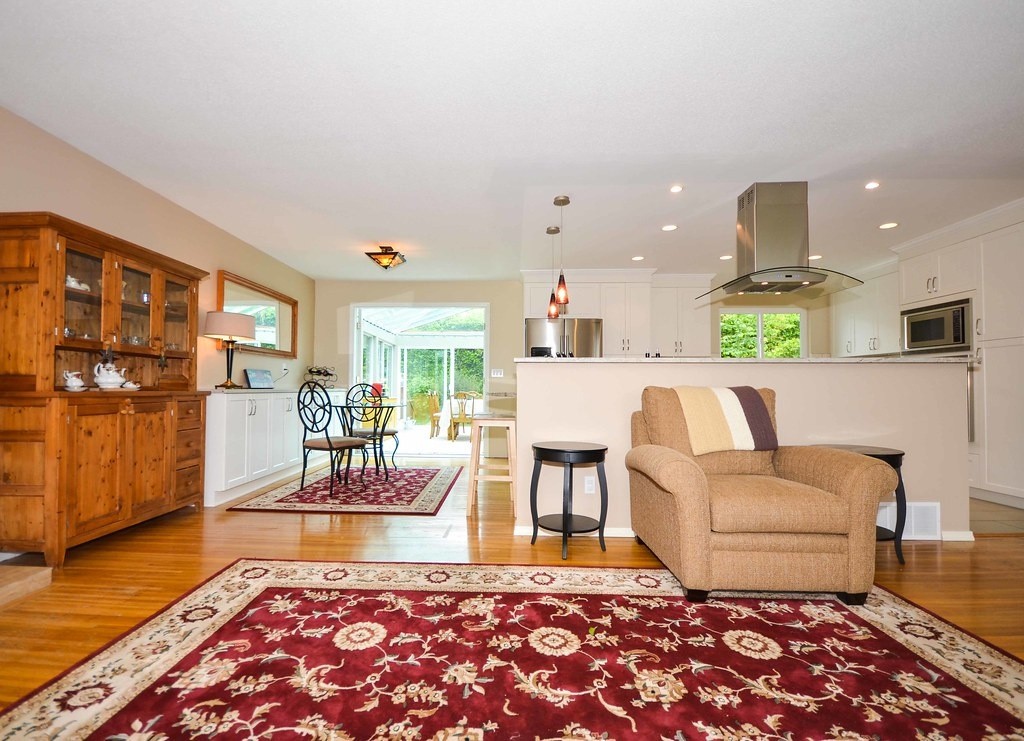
[141,289,150,305]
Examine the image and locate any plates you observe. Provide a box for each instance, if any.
[63,387,88,392]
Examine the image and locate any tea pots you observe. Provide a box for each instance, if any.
[93,362,127,388]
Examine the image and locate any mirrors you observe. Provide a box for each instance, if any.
[216,270,298,359]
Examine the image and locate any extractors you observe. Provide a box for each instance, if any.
[695,182,865,301]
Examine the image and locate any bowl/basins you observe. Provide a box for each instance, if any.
[65,328,77,339]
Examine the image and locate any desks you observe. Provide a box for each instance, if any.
[438,399,483,438]
[319,403,407,482]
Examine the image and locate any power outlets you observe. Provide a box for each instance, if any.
[282,363,287,372]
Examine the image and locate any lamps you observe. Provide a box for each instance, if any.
[364,246,407,270]
[203,310,256,389]
[555,195,570,305]
[547,226,560,318]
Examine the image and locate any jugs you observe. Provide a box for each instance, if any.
[63,370,85,387]
[66,275,80,289]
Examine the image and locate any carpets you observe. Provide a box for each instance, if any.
[0,557,1024,741]
[225,464,463,516]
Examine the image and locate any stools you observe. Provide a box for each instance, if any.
[466,413,516,518]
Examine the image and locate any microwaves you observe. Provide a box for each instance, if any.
[907,308,964,349]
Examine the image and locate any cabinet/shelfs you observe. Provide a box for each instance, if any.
[975,337,1024,500]
[973,221,1024,343]
[651,287,711,357]
[901,236,981,310]
[0,212,213,570]
[832,286,854,358]
[600,282,650,358]
[205,391,347,507]
[854,271,902,356]
[524,282,600,318]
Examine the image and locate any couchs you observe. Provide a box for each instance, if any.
[625,384,901,607]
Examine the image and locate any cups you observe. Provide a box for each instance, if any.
[120,335,180,350]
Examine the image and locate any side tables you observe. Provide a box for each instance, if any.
[810,444,908,566]
[529,441,608,561]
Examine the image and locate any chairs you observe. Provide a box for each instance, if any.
[297,380,401,499]
[466,391,483,401]
[449,390,475,443]
[411,391,442,439]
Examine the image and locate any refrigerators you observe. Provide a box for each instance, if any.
[524,318,603,357]
